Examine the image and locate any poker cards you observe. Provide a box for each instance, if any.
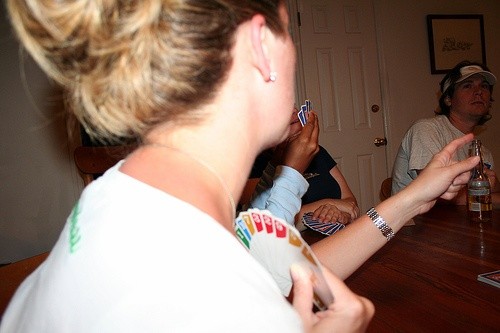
[234,208,346,311]
[477,270,500,288]
[298,99,313,128]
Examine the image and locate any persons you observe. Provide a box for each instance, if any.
[235,100,361,231]
[0,1,480,333]
[391,61,500,205]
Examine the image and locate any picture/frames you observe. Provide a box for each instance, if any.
[427,14,487,75]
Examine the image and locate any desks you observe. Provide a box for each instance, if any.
[301,204,500,333]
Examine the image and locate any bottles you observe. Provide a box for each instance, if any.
[467,140,492,223]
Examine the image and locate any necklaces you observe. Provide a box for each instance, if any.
[141,141,237,228]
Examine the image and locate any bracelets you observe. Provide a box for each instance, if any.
[365,207,396,241]
